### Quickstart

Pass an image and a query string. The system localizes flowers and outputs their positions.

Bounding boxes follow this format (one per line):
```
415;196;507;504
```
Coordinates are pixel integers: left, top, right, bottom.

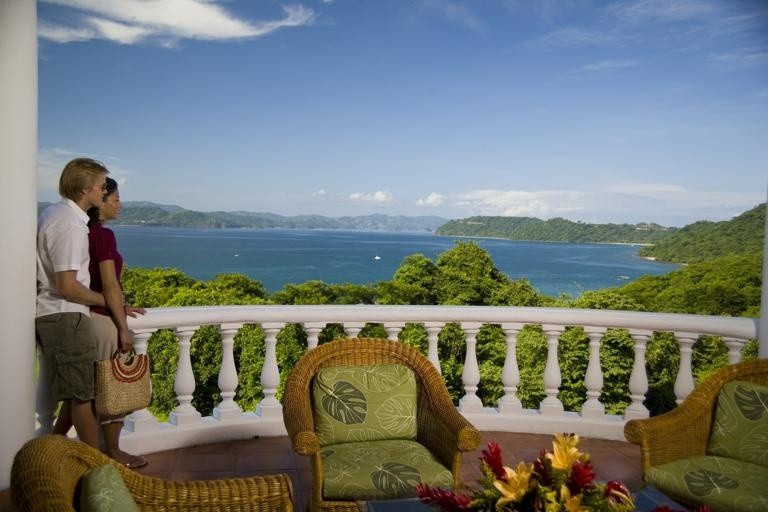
416;430;639;512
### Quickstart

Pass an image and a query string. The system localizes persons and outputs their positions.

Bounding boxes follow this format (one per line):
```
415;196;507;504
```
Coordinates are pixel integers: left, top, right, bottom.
35;157;129;450
86;174;149;470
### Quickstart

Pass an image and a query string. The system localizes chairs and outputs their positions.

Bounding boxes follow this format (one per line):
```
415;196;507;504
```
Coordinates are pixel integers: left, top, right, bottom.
11;435;295;511
625;358;766;512
283;336;482;506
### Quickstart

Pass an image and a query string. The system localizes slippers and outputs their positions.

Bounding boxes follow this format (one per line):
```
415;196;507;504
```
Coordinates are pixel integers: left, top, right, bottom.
110;454;149;469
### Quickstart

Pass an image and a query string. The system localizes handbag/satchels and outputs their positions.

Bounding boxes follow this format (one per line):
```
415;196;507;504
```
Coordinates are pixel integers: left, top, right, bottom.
93;346;153;417
